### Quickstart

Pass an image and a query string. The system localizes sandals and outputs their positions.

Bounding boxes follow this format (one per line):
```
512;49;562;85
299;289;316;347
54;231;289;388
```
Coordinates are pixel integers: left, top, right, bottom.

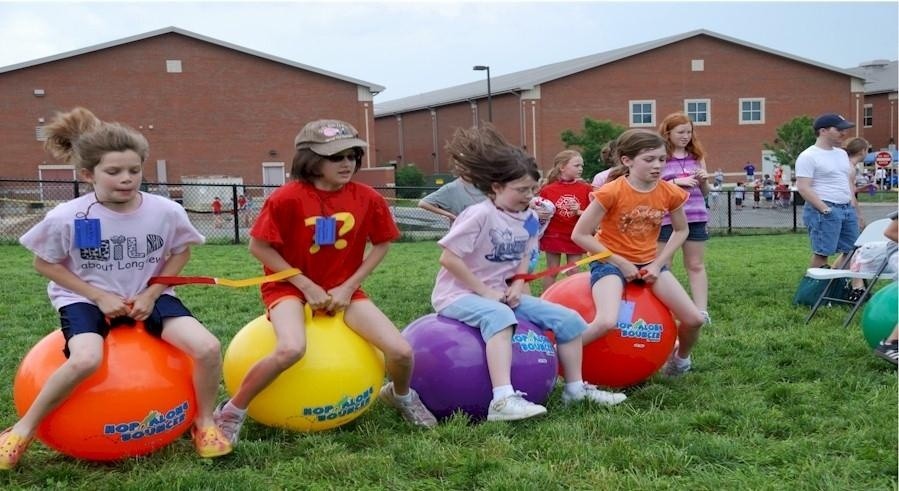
0;426;38;473
186;420;233;460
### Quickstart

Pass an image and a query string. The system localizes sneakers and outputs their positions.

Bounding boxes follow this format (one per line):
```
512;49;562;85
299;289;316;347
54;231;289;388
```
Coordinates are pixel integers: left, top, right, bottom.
664;340;692;379
700;311;713;329
485;391;548;423
847;286;870;304
874;333;898;367
377;382;439;430
559;381;628;410
212;399;250;446
735;206;762;210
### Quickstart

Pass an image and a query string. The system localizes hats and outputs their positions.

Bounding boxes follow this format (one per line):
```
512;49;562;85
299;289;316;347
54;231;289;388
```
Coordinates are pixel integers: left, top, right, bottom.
812;113;857;131
293;119;369;159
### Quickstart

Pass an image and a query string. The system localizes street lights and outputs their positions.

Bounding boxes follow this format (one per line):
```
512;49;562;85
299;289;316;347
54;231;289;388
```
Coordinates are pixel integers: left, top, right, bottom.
388;160;399;199
472;64;495;128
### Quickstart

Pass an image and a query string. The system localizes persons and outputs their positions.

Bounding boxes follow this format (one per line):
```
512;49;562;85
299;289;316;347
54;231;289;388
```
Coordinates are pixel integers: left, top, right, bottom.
209;194;254;231
0;106;233;470
539;149;596;291
592;139;630;188
212;118;440;451
430;121;628;422
554;130;705;379
418;155;490;228
656;112;713;324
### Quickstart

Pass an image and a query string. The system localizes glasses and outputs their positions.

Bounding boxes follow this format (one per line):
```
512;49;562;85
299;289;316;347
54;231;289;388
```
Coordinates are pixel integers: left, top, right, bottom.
502;183;541;198
318;151;360;165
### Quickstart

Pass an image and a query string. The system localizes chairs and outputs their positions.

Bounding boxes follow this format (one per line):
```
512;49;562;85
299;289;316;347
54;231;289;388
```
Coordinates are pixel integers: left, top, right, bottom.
804;219;899;330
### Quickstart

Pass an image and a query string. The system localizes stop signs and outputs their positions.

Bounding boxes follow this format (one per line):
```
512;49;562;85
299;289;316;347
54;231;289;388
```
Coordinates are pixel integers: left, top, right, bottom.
876;151;893;170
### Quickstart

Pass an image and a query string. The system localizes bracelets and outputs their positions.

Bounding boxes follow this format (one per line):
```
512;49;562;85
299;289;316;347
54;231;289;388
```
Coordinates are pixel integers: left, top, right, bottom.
670;177;676;185
